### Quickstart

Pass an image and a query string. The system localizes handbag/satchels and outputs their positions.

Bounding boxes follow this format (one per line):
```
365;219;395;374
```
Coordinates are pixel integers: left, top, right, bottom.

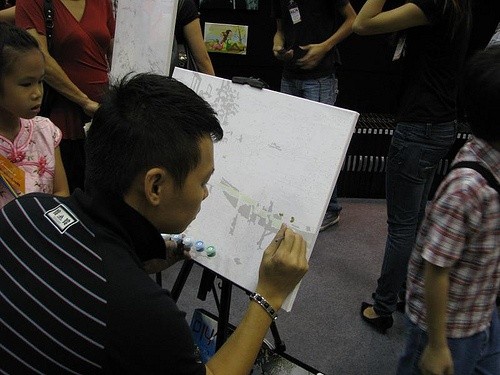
172;44;197;77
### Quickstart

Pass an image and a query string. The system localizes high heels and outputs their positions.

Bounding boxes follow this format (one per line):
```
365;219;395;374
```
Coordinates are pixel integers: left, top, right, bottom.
373;292;406;313
360;301;394;333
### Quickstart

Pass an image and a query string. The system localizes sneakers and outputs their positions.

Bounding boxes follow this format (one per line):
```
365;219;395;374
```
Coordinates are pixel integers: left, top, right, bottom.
319;209;339;231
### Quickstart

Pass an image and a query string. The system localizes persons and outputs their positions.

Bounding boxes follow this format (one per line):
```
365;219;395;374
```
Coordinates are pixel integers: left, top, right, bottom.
14;0;116;194
0;4;15;23
0;22;73;211
168;0;216;78
352;1;473;334
0;69;309;375
395;46;500;375
272;0;357;231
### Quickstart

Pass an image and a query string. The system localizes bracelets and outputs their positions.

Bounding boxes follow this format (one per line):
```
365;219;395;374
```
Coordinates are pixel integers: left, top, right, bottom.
249;292;279;323
